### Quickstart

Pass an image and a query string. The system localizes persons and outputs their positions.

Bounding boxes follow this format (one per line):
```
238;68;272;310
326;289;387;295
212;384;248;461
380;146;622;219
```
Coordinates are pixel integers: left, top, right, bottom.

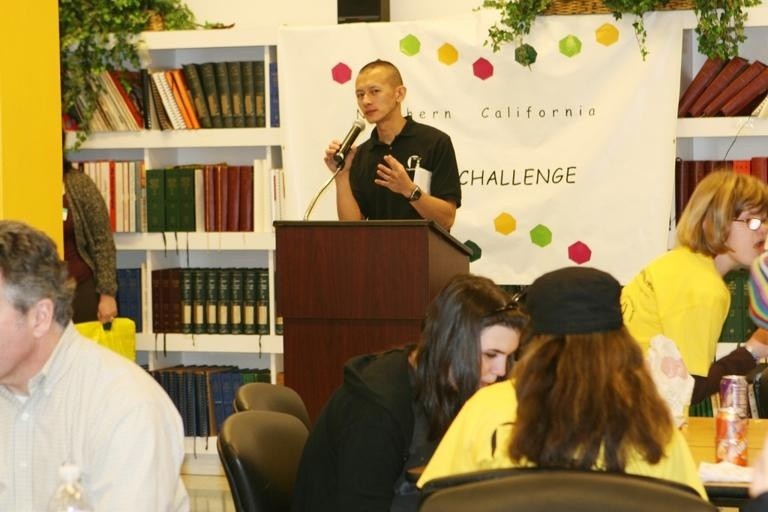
323;60;461;234
62;159;118;331
415;268;710;506
618;172;768;433
0;218;189;512
292;273;526;512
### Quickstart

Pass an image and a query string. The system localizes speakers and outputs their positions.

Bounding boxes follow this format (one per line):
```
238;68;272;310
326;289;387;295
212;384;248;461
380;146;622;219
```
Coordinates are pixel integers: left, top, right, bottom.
338;0;390;24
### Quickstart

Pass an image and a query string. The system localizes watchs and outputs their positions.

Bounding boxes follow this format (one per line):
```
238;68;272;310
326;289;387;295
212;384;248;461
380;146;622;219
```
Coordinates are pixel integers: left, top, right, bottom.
404;184;422;202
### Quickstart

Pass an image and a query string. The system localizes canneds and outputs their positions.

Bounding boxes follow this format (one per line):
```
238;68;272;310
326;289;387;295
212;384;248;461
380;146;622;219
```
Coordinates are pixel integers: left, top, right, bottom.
714;409;750;468
721;374;747;410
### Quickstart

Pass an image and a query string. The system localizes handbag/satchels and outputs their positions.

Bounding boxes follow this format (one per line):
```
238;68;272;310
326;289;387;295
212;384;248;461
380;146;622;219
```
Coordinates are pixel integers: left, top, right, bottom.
74;318;136;361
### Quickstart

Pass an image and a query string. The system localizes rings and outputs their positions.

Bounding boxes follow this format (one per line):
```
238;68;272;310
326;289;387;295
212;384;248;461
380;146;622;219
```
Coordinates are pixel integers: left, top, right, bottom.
110;317;114;320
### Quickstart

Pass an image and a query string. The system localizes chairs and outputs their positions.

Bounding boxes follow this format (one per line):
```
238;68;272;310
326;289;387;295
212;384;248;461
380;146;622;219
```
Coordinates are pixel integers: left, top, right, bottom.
229;381;316;437
215;410;311;511
415;466;722;511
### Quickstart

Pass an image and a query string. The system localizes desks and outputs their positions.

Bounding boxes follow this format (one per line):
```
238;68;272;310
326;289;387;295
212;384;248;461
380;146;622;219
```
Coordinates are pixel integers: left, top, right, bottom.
406;413;768;508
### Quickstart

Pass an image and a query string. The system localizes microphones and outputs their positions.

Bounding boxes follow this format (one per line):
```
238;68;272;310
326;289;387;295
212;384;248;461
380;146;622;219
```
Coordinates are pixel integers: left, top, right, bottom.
334;120;366;167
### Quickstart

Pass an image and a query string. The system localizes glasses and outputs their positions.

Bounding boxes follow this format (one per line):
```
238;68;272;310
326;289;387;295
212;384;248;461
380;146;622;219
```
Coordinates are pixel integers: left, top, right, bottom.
733;215;765;231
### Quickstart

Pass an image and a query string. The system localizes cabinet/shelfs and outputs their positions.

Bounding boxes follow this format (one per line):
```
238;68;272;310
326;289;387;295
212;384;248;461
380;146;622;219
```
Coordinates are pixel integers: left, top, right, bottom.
62;27;281;474
669;7;768;418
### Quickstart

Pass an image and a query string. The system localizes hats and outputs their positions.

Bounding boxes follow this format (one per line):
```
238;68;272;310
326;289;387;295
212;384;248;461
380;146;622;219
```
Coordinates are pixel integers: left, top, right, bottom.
525;267;624;334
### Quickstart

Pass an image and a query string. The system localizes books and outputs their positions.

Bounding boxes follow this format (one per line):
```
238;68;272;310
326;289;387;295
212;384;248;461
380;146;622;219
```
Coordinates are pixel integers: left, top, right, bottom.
677;57;768;420
141;364;270;437
62;60;266;134
118;267;267;335
61;157;268;232
